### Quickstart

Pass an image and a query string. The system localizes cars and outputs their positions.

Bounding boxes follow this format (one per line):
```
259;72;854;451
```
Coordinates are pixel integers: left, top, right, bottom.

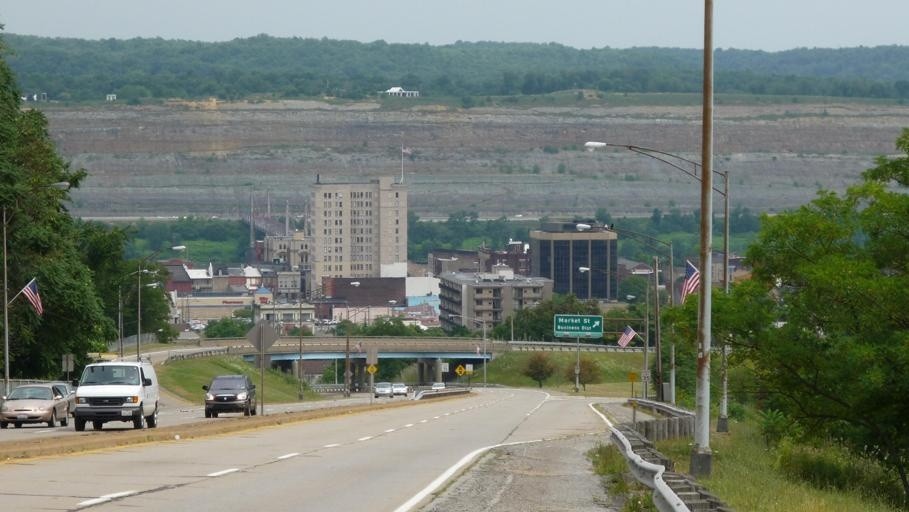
373;382;394;398
202;375;255;418
392;383;408;396
0;383;76;428
431;383;445;390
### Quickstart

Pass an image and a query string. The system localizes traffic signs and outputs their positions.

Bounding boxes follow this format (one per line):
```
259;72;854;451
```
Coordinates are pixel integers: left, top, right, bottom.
553;314;604;339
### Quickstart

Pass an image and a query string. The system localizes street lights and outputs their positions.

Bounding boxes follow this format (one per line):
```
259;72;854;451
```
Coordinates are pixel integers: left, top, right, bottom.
299;281;360;400
119;245;185;362
585;141;729;432
448;314;486;389
344;300;397;398
576;223;675;405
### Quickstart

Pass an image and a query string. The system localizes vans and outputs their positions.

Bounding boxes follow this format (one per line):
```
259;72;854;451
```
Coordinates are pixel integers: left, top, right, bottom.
74;362;159;431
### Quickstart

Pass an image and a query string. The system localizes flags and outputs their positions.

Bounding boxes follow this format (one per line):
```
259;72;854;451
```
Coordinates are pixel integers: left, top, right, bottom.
22;281;43;316
618;327;636;348
680;262;700;305
